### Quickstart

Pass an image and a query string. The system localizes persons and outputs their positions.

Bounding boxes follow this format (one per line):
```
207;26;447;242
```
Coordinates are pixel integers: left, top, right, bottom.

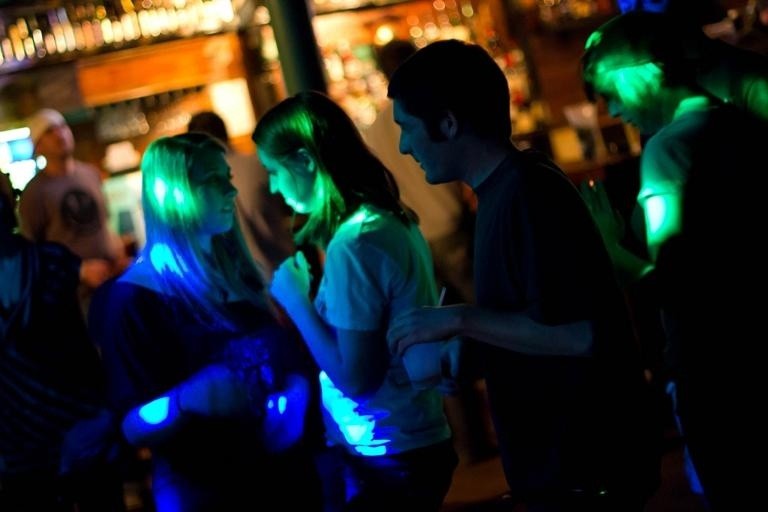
382;39;668;510
88;130;350;512
1;190;130;511
577;8;768;510
13;107;118;266
251;91;459;510
185;109;278;325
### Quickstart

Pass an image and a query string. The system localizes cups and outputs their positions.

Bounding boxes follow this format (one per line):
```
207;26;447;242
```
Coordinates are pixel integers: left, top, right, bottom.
402;340;443;392
